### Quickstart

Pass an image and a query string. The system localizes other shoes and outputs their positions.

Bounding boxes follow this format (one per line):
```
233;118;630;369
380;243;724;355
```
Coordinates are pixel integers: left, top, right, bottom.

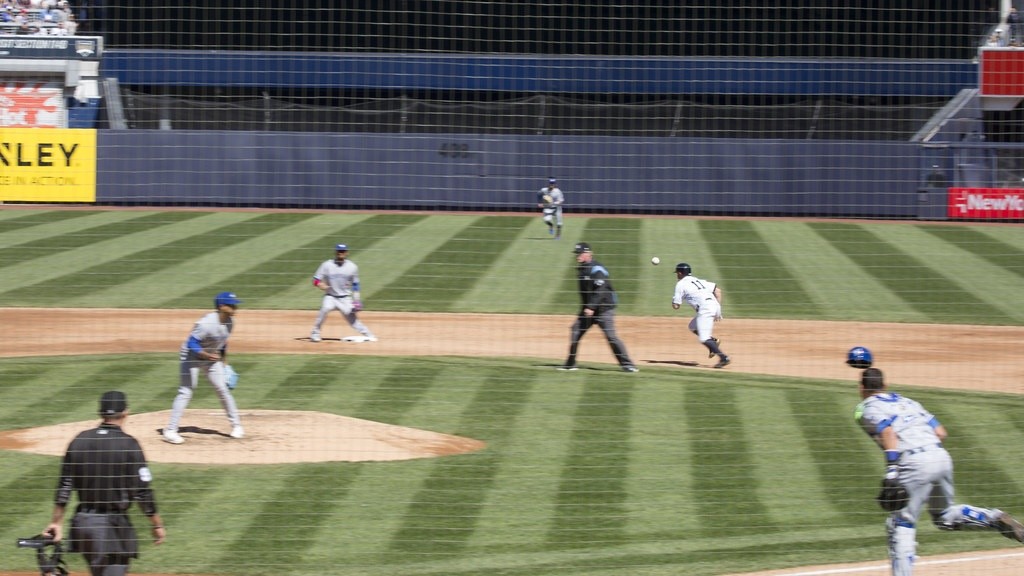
556;362;579;371
997;514;1024;542
366;336;377;342
715;356;730;369
626;367;639;372
709;338;720;359
311;330;321;342
230;426;245;439
162;428;185;443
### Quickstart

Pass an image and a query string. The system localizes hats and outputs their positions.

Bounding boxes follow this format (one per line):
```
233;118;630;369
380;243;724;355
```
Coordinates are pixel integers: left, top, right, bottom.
337;245;347;251
549;177;557;183
100;391;125;415
215;291;241;305
673;264;691;273
571;242;593;252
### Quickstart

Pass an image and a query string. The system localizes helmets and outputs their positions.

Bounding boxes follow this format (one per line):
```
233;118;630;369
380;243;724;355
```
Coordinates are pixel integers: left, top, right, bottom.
846;347;871;367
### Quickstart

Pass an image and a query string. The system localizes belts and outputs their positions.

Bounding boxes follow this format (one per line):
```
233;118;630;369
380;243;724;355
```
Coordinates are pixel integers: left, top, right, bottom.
908;442;943;455
696;298;713;312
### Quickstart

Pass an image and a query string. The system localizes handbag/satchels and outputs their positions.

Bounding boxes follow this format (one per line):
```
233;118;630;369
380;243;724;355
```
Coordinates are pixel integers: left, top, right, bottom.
106;515;138;559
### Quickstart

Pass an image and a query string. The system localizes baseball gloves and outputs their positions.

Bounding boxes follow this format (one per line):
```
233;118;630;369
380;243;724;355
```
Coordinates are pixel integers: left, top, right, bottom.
225;364;238;391
878;479;910;511
543;194;554;204
15;534;62;572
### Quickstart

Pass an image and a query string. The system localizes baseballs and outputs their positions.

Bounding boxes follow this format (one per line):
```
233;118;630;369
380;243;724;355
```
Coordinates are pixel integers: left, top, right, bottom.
652;257;660;264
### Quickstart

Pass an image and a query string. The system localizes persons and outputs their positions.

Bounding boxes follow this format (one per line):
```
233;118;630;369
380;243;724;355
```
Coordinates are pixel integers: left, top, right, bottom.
672;263;731;369
559;242;639;372
987;7;1024;48
0;0;78;37
40;391;167;576
537;176;565;240
854;367;1024;576
162;292;244;444
310;243;377;342
